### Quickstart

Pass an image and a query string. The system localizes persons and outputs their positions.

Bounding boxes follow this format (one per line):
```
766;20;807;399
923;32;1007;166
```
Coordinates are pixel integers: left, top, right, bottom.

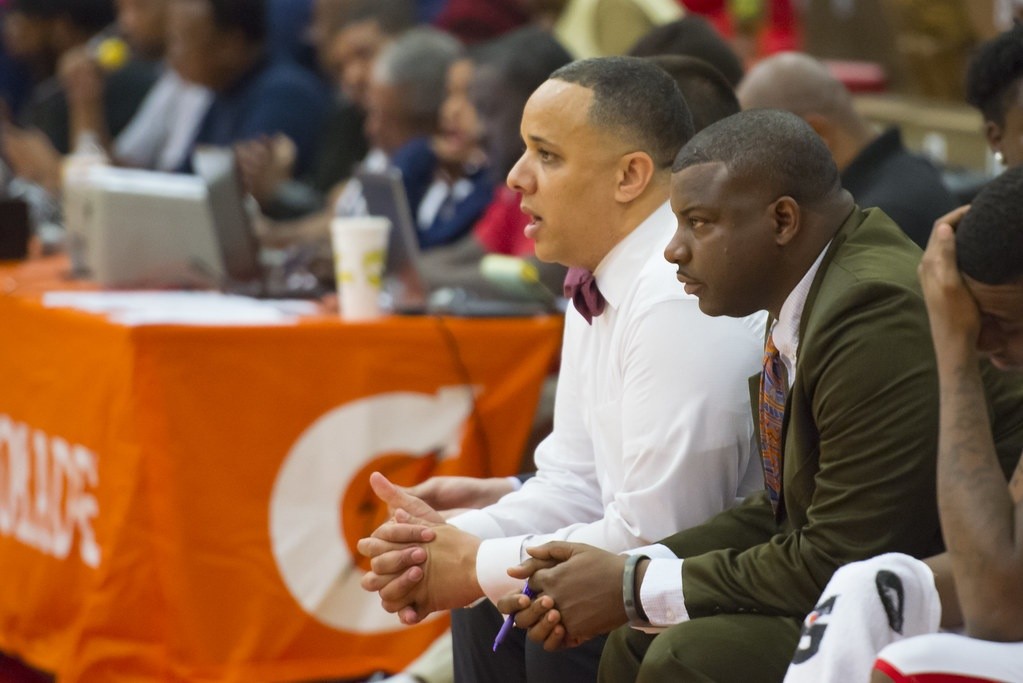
781;165;1023;683
736;52;957;251
968;26;1023;168
357;55;769;683
629;20;747;86
496;104;1023;683
0;0;573;313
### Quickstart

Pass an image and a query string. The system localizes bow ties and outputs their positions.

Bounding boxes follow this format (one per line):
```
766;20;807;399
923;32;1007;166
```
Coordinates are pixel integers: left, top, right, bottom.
563;267;606;325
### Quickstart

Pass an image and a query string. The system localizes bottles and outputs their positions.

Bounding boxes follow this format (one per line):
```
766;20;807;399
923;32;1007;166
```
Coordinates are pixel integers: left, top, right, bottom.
62;129;108;277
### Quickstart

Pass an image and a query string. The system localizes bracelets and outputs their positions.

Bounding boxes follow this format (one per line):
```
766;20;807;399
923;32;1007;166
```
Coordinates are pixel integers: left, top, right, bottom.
622;555;650;626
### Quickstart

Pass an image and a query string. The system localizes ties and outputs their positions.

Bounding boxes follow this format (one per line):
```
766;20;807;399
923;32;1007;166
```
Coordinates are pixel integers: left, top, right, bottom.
759;320;793;526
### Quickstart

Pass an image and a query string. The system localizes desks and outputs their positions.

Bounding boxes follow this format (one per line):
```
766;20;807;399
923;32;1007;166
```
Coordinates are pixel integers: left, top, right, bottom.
2;256;561;683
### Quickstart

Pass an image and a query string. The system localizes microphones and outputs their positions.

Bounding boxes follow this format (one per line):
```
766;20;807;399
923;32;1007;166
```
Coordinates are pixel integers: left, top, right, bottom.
32;38;132;105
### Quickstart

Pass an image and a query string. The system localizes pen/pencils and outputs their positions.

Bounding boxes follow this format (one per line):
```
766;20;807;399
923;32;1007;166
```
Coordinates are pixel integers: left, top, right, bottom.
492;583;535;652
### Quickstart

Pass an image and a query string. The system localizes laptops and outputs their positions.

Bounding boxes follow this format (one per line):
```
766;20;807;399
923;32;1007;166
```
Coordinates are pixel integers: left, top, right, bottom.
354;163;552;314
196;144;329;297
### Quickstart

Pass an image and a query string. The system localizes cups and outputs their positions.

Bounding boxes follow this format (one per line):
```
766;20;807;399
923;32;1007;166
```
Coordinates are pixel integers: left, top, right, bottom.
327;215;393;324
